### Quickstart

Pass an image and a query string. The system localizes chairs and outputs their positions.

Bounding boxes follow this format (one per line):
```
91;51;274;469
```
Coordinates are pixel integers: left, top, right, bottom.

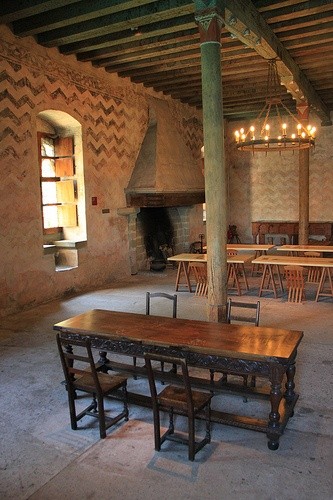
210;297;260;403
144;353;214;462
188;262;207;297
283;266;306;304
305;252;321;284
56;332;129;440
133;291;178;386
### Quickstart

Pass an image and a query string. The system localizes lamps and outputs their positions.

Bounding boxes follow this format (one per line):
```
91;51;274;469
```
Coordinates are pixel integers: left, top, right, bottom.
234;58;317;155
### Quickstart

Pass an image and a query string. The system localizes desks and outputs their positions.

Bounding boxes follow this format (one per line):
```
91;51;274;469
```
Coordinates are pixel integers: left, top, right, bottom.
53;308;304;450
167;244;333;302
